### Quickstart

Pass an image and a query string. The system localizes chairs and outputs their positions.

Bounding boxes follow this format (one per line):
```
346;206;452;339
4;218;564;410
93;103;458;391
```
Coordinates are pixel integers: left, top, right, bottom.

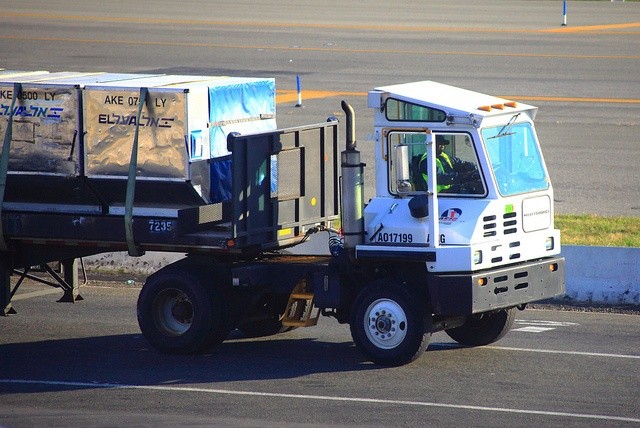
411;155;426;192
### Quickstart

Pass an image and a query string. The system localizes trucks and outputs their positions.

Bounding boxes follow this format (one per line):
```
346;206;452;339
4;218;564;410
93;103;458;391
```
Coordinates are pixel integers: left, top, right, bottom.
0;68;566;367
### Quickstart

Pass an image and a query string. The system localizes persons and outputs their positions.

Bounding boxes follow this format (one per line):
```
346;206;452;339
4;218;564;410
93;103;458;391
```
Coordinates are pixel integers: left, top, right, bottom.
418;136;471;194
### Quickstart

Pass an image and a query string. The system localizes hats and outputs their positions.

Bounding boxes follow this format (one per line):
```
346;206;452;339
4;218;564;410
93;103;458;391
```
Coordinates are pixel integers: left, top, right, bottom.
437;136;449;145
